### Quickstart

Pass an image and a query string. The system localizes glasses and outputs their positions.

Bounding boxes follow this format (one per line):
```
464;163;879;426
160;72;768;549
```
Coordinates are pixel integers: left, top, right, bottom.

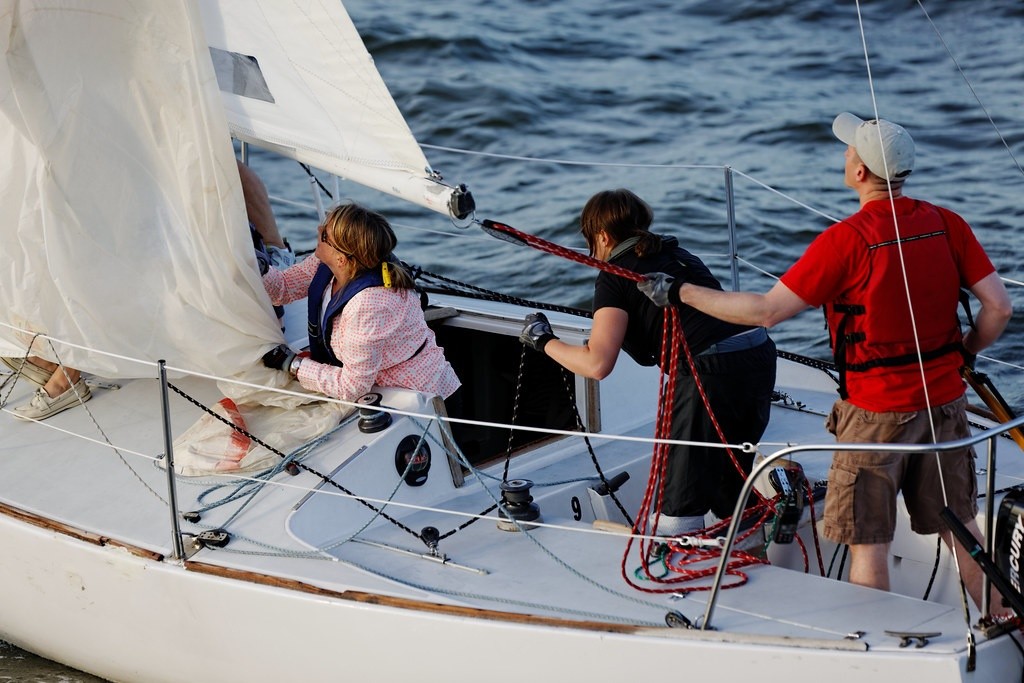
322;222;353;258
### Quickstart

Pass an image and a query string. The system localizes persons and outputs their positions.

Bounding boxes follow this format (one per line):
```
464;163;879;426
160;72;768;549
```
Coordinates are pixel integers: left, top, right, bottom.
632;111;1014;621
256;201;482;466
236;155;290;338
516;188;777;564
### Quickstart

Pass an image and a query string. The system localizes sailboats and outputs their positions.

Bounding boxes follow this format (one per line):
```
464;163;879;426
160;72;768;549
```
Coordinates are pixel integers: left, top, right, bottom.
0;2;1024;679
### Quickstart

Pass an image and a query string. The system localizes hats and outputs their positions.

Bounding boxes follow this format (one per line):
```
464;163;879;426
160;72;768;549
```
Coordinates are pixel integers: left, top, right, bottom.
832;112;914;182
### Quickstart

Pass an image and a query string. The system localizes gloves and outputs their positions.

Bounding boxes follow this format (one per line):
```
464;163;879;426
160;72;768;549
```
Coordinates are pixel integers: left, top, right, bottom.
520;312;560;351
637;273;682;305
262;345;297;373
955;336;976;370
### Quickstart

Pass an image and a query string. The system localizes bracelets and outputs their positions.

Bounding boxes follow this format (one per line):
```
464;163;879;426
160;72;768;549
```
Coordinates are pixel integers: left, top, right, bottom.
290;355;303;375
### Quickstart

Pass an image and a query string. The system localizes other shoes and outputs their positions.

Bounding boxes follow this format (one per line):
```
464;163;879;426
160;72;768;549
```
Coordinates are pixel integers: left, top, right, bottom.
13;377;92;421
1;357;52;388
